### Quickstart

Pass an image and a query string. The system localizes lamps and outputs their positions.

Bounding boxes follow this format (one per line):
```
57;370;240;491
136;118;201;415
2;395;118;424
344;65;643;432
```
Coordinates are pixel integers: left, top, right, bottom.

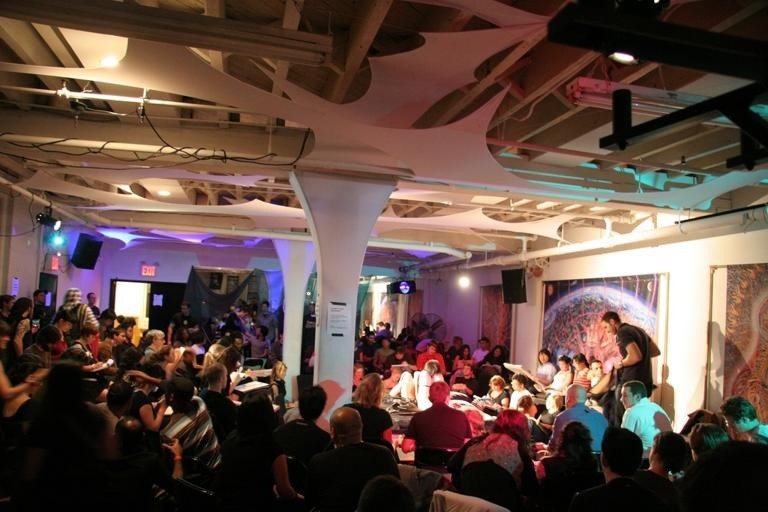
36;199;64;233
0;0;336;71
558;72;766;131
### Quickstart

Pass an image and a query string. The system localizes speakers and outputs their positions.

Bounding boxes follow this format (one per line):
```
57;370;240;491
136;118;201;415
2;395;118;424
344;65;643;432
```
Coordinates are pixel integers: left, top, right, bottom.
70;233;104;270
208;271;224;290
501;268;527;304
390;280;416;295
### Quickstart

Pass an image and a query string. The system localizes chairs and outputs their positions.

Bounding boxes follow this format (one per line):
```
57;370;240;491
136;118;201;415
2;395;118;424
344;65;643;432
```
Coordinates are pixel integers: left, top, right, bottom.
10;319;650;511
